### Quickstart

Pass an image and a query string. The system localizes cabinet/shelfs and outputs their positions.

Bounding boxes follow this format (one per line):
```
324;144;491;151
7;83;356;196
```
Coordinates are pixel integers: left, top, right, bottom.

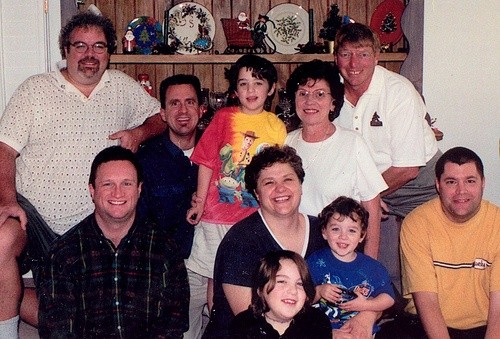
60;0;424;113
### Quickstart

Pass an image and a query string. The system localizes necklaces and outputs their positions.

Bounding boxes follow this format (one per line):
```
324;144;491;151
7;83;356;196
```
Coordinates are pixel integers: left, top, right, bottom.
265;314;291;323
296;126;329;170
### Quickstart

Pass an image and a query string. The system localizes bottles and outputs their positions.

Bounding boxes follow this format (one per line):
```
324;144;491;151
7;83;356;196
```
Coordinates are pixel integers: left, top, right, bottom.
125;26;136;54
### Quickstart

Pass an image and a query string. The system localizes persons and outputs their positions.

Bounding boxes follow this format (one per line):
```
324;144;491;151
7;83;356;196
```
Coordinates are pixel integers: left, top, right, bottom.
0;13;167;339
213;145;406;339
37;146;190;339
227;250;332;339
399;147;500;339
331;23;443;296
134;74;208;339
283;59;389;260
306;196;395;339
186;55;287;315
252;14;268;53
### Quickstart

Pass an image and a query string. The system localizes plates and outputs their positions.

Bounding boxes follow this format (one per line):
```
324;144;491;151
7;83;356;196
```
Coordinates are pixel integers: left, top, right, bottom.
261;3;309;54
125;16;163;55
162;2;216;55
370;0;405;46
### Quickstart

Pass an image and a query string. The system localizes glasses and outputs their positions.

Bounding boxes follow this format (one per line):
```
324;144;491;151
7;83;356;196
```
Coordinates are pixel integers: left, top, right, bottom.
295;90;332;99
69;41;109;54
